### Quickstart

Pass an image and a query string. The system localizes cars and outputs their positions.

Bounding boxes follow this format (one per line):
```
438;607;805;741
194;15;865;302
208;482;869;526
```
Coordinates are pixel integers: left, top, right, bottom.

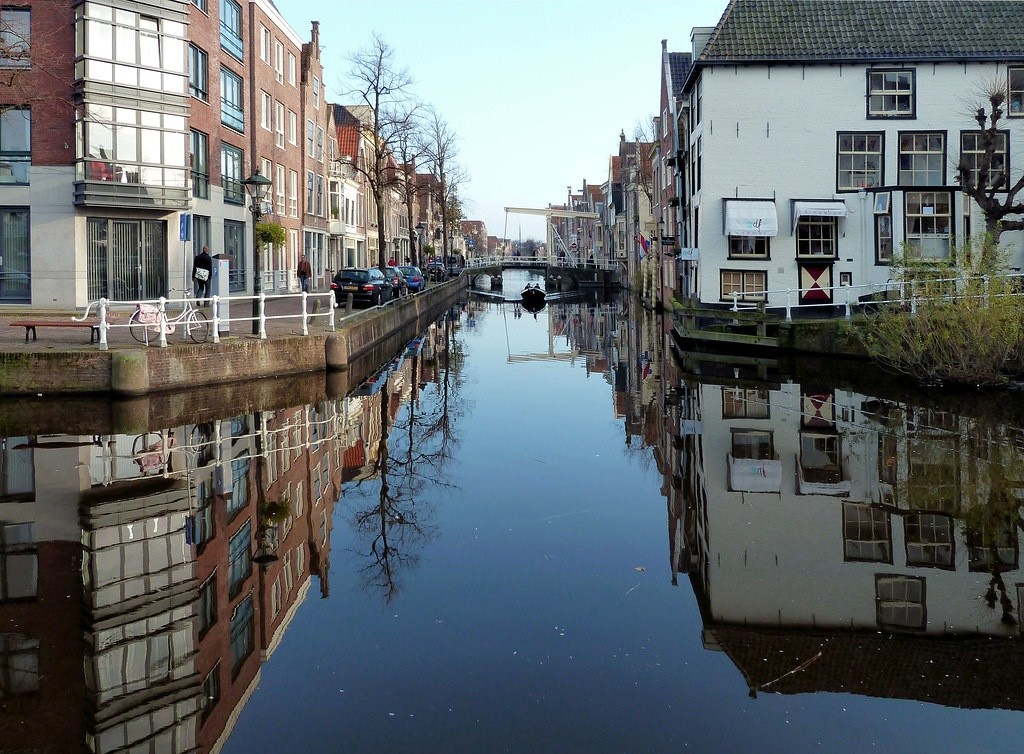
399;266;424;293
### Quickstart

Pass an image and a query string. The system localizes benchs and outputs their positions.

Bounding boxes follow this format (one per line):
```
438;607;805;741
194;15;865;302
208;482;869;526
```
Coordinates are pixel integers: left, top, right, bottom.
8;320;115;344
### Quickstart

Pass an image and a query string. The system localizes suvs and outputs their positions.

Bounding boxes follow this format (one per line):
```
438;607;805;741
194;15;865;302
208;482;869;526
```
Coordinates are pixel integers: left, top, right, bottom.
427;263;447;282
330;265;393;308
377;267;408;299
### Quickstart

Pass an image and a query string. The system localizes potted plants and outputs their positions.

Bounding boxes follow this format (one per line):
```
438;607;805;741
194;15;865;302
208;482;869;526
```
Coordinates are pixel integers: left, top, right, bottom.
667;197;679;207
331;206;338;218
254;220;287;254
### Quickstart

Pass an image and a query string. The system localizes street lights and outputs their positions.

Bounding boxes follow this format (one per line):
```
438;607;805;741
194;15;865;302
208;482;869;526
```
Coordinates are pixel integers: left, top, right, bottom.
244;166;272;334
415;221;424;270
247;412;279;573
448;236;454;278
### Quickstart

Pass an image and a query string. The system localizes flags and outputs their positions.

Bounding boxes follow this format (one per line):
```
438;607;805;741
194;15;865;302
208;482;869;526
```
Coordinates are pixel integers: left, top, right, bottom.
639;235;650;260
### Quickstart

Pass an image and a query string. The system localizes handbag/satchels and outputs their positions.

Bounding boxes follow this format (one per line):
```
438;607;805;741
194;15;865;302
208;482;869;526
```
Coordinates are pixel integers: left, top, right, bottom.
195;267;210;281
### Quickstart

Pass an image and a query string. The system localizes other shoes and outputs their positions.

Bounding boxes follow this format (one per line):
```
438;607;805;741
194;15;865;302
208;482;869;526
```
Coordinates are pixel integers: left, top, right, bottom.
197;301;200;306
204;304;210;308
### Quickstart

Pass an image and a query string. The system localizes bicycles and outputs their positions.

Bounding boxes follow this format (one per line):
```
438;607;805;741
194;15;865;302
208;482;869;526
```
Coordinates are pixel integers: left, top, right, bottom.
128;287;208;345
132;422;212;474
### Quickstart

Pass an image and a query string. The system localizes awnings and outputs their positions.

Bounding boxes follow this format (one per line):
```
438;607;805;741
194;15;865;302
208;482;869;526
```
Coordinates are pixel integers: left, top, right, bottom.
792;201;847;237
725;200;777;237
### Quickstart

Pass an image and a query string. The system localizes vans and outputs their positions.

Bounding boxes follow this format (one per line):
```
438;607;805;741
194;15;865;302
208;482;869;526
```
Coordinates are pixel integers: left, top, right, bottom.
443;256;460;274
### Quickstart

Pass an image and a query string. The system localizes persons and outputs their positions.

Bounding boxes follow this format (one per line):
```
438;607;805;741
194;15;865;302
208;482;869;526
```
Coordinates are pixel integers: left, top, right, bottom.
405;256;411;266
427;256;442;263
557;248;565;267
192;246;213;307
298;255;312;293
512;249;521;266
532;249;536;265
371;260;378;267
388;257;396;267
